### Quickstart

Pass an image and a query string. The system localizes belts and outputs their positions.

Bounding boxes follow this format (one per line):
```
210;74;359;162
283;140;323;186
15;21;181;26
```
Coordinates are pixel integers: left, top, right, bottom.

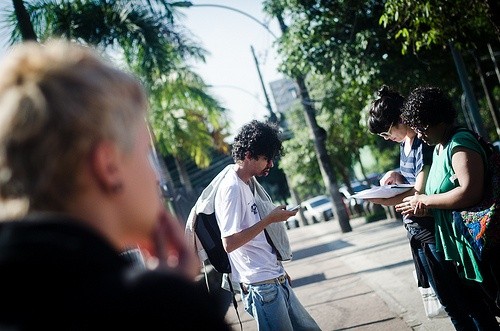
251;275;286;285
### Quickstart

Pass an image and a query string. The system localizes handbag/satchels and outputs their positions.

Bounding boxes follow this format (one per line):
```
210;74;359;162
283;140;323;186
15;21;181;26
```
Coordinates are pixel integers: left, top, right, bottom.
413;269;448;320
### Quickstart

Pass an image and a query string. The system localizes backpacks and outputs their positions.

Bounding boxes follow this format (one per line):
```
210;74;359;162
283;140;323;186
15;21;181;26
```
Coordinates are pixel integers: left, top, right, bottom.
447;129;500;255
194;213;243;331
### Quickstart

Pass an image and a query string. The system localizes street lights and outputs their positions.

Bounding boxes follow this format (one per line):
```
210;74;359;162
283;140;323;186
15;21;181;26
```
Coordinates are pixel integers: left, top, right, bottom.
171;1;282;42
282;177;385;229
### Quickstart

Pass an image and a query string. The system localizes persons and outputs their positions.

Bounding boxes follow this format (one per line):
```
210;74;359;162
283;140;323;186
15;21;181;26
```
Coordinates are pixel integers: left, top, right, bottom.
0;37;233;331
213;121;323;331
361;85;500;331
395;87;500;331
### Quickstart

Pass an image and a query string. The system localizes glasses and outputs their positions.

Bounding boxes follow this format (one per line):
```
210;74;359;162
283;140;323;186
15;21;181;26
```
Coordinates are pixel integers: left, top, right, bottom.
377;121;393;137
414;123;430;133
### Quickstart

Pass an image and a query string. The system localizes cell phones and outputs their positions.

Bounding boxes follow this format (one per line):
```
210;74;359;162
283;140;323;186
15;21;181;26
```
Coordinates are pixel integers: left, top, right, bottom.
287;204;301;211
